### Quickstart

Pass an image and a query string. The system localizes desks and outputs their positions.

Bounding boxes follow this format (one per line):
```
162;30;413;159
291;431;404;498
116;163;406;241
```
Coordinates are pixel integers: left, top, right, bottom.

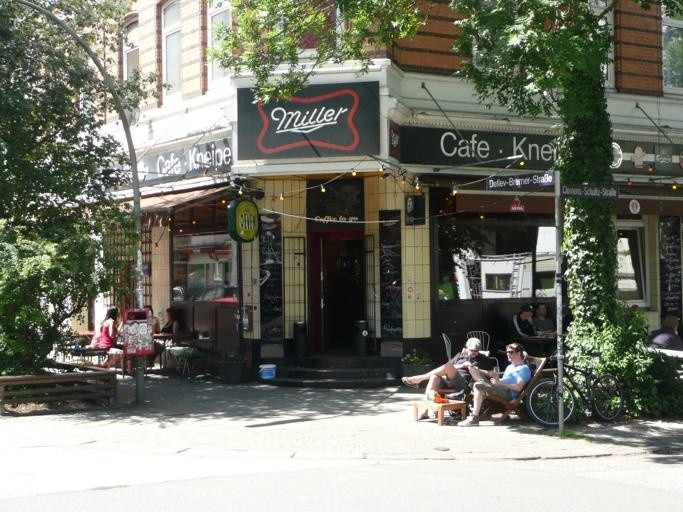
153;331;173;369
416;397;468;424
513;335;555;353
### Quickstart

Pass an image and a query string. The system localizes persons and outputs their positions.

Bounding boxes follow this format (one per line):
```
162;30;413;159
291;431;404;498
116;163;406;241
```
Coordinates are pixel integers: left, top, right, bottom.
514;304;541;356
647;316;683;351
145;308;178;366
401;338;493;420
626;305;640;324
456;344;531;426
91;309;135;375
144;305;160;332
531;304;557;336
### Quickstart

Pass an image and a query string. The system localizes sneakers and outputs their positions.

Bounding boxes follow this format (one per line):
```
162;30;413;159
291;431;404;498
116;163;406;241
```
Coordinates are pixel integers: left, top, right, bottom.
458;413;479;426
474;379;492;391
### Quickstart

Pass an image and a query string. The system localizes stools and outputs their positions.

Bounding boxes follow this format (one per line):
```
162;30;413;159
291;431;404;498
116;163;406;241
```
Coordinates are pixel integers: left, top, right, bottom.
172;347;199;376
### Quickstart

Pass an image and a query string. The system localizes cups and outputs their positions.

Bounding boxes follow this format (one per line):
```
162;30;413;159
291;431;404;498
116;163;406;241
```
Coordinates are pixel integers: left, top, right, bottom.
494;367;499;380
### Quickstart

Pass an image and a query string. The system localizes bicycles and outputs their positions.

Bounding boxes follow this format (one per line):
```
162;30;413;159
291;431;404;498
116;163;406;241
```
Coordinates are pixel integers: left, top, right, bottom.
525;347;624;428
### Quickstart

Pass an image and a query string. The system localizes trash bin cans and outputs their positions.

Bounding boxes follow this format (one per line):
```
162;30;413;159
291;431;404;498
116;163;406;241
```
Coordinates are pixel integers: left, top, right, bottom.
293;320;306;357
354;320;368;357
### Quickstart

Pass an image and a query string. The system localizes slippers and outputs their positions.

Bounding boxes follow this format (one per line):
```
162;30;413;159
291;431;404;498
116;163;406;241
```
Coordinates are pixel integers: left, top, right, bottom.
418;414;429;420
401;377;419;389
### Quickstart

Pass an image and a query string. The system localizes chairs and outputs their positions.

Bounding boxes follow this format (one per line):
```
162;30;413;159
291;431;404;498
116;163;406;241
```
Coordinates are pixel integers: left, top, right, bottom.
442;330;547;421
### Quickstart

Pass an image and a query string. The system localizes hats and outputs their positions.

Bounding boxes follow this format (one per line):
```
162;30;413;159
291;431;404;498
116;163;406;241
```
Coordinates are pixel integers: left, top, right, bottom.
520;304;536;312
466;338;481;351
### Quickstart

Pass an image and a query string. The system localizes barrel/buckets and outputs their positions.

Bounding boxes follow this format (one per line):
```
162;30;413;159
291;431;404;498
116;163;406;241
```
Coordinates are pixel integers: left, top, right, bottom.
258;364;276;379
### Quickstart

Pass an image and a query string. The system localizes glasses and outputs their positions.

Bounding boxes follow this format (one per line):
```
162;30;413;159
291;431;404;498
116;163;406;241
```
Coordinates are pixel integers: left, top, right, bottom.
506;351;513;354
